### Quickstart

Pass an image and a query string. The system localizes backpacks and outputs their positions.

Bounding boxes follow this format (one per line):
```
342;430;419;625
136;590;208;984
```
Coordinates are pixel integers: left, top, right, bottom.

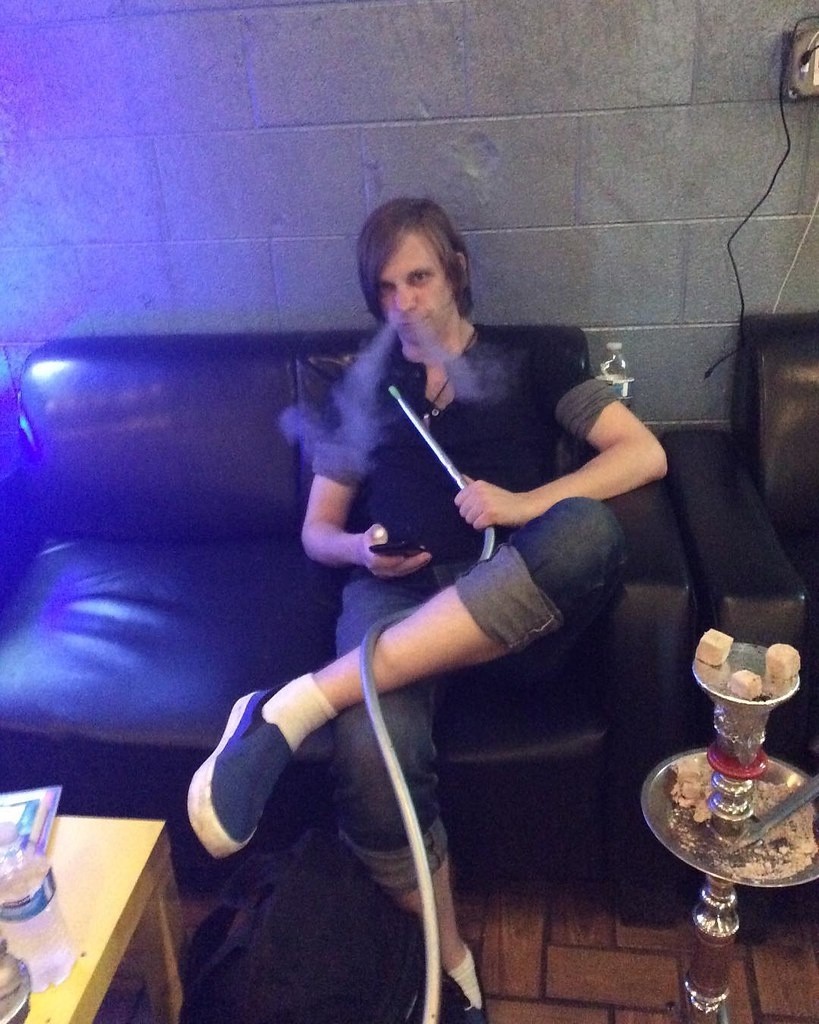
178;838;466;1024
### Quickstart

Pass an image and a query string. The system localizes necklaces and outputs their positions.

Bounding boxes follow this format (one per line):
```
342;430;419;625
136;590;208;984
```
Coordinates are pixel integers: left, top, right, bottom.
422;329;478;431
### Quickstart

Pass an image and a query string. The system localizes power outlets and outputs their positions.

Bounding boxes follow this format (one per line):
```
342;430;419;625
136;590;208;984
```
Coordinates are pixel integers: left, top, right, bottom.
787;27;819;100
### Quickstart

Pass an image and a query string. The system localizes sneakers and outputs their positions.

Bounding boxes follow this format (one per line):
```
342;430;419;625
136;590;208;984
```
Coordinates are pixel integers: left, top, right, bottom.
187;683;293;858
454;947;486;1024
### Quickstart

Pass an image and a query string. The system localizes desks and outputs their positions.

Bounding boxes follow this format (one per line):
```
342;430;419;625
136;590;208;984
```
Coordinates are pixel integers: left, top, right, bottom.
1;815;191;1024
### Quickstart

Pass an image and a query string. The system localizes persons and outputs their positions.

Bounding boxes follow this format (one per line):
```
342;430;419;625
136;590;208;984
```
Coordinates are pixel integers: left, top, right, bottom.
186;198;668;1024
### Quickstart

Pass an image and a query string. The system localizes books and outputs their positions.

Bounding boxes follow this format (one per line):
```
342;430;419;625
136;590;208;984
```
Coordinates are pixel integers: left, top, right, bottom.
0;783;62;856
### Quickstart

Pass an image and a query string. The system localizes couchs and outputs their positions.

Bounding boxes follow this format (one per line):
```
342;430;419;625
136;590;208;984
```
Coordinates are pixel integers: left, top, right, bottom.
0;327;691;923
659;312;818;917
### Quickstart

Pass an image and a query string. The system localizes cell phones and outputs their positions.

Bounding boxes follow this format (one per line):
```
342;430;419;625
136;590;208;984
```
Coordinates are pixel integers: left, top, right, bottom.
369;540;426;556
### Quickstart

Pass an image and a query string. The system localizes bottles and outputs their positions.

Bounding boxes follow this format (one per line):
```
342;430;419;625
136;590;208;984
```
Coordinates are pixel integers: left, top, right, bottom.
595;342;635;412
0;823;73;995
